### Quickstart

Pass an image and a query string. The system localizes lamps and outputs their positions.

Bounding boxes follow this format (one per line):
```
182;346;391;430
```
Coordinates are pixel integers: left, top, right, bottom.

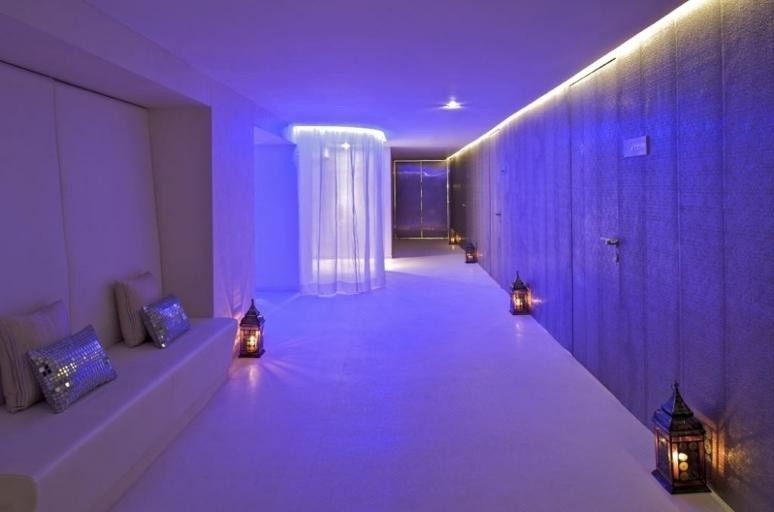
509;270;530;316
448;228;458;245
650;379;710;496
464;241;477;265
237;297;266;360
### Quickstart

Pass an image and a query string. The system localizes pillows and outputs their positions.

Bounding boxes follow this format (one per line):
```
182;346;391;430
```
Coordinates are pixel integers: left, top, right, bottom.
0;296;73;414
138;293;191;351
24;324;117;413
112;270;162;348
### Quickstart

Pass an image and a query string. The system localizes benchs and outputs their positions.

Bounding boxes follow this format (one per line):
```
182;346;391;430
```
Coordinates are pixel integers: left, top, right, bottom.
0;314;236;512
